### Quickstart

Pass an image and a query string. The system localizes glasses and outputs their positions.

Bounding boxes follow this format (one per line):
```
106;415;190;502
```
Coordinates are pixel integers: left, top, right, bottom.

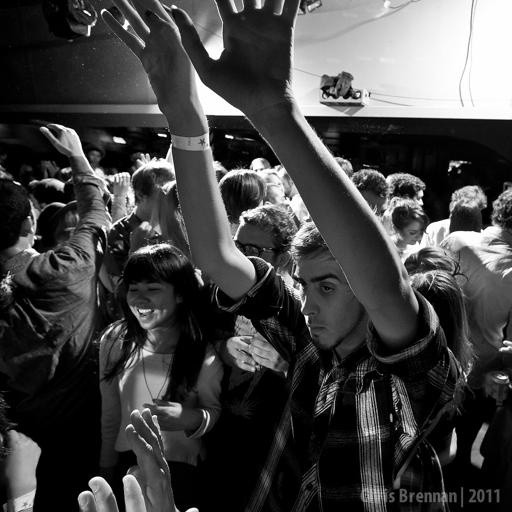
233;240;275;257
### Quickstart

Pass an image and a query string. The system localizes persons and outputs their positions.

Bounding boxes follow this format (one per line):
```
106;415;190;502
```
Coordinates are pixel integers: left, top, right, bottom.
98;0;466;512
